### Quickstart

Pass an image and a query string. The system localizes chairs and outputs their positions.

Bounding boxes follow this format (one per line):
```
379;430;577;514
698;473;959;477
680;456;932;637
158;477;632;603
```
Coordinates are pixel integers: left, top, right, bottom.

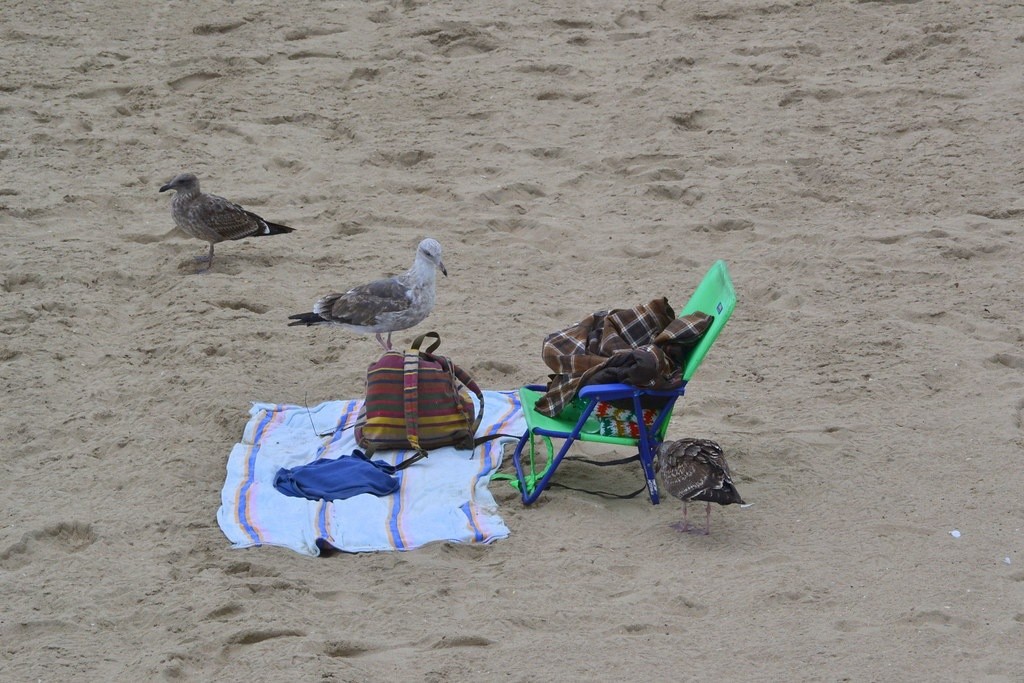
512;260;739;510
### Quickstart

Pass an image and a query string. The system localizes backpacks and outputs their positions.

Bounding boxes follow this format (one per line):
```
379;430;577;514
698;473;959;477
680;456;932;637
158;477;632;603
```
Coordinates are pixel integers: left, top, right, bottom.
353;331;523;460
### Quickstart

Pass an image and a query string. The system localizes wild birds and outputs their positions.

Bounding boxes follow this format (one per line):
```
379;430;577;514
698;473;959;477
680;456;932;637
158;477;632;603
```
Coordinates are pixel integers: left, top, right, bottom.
287;238;449;351
160;171;295;272
656;435;746;538
